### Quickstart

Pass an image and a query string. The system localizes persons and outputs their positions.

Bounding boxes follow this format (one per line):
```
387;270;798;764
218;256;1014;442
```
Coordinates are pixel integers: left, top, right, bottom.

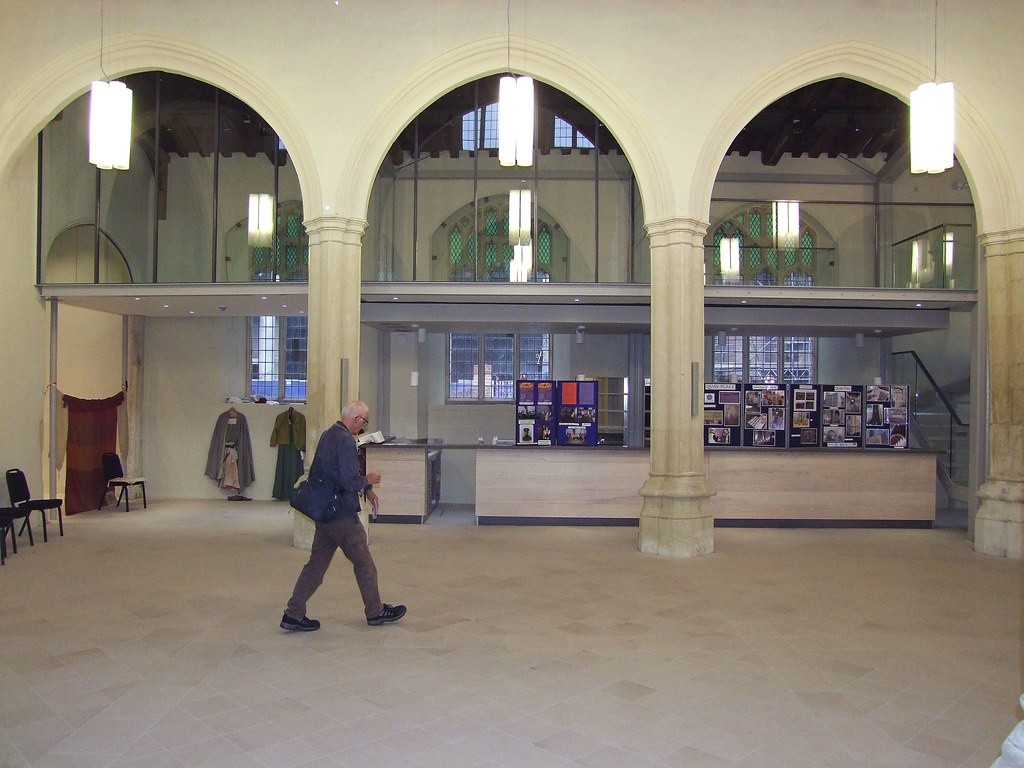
753;392;760;407
713;433;727;442
522;428;531;441
280;400;407;632
763;391;784;405
543;427;550;439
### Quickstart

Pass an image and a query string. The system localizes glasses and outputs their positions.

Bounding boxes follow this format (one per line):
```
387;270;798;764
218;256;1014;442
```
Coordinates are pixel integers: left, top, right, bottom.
356;415;369;426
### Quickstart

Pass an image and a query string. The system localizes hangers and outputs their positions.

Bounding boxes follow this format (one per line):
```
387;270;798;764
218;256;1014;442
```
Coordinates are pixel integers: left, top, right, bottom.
278;402;307;419
219;400;247;420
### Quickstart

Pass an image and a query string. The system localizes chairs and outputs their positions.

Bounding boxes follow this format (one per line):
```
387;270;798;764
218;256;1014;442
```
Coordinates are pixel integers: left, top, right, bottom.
0;468;63;565
98;452;147;513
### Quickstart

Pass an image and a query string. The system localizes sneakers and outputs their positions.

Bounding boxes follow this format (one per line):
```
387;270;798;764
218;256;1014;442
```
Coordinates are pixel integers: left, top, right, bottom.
280;614;320;631
367;604;407;626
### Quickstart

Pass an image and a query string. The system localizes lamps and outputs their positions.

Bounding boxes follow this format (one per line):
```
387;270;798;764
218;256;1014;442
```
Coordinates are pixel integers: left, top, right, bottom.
514;237;533;271
510;259;528;282
248;193;276;249
88;77;133;171
874;330;882;386
718;330;727;347
910;0;956;175
855;333;865;348
497;0;535;247
418;328;426;343
576;329;584;344
410;324;419;387
720;201;801;272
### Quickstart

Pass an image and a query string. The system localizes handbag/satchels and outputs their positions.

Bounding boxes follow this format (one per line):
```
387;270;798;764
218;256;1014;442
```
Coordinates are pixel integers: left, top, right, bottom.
290;476;341;524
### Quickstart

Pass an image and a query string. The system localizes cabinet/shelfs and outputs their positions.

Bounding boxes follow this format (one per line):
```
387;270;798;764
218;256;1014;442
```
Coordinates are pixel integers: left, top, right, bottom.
362;434;441;524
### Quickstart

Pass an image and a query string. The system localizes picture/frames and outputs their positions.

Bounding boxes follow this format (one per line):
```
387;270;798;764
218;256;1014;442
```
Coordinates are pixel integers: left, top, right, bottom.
704;385;908;448
